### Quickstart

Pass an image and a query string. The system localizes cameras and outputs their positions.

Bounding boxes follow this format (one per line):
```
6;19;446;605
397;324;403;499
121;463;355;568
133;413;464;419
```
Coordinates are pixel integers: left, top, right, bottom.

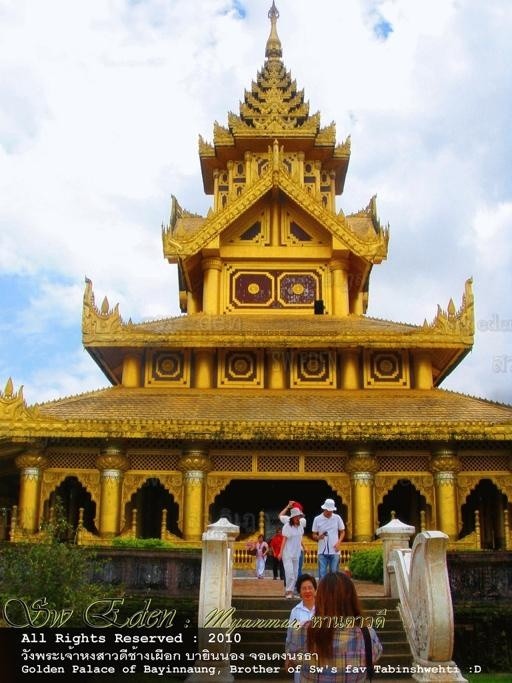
323;531;329;536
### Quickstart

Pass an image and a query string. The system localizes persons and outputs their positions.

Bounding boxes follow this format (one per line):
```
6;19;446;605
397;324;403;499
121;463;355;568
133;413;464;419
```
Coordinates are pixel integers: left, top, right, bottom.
285;570;383;678
256;499;345;590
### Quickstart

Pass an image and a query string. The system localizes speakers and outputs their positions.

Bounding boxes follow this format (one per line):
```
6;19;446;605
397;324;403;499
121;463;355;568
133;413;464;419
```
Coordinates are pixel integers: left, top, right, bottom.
314;300;323;314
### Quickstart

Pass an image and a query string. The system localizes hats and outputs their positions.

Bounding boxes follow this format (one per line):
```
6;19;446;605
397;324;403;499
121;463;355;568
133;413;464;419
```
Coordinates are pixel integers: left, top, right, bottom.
288;503;306;519
320;498;337;511
